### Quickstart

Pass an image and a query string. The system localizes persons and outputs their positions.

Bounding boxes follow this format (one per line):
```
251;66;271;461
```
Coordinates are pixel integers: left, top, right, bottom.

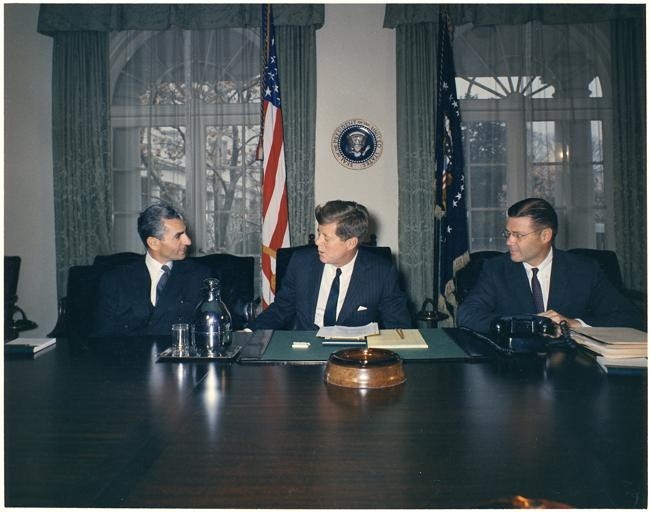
88;203;214;337
236;200;413;333
455;197;644;332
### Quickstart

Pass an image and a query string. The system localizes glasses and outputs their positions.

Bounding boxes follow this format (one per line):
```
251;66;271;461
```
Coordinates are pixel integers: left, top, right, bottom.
503;227;539;240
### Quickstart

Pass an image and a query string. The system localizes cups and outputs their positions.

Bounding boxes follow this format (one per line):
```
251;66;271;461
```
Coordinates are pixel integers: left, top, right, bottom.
171;323;190;357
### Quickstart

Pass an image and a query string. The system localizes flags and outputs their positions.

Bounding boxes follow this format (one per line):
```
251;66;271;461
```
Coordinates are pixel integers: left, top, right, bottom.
256;4;291;310
433;5;471;329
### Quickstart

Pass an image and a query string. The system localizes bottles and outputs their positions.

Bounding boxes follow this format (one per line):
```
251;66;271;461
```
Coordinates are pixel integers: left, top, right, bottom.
190;278;233;358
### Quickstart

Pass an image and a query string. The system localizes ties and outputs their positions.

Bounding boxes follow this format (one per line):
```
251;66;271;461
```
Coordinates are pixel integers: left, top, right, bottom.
323;269;342;326
155;266;170;304
530;268;544;314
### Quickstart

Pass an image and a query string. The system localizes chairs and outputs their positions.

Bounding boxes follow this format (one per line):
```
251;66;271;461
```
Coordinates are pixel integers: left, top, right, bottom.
2;255;40;339
191;253;261;320
275;244;393;298
453;250;508;305
564;247;646;305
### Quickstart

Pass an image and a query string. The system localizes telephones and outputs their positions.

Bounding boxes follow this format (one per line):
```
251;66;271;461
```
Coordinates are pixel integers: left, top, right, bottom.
489;314;555;357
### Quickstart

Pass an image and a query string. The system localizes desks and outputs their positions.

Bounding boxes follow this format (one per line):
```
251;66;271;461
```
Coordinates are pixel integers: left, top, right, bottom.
4;330;650;508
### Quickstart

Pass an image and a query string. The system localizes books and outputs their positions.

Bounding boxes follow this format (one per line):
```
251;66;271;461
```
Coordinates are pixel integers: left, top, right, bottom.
316;322;429;350
4;336;57;354
571;325;647;366
